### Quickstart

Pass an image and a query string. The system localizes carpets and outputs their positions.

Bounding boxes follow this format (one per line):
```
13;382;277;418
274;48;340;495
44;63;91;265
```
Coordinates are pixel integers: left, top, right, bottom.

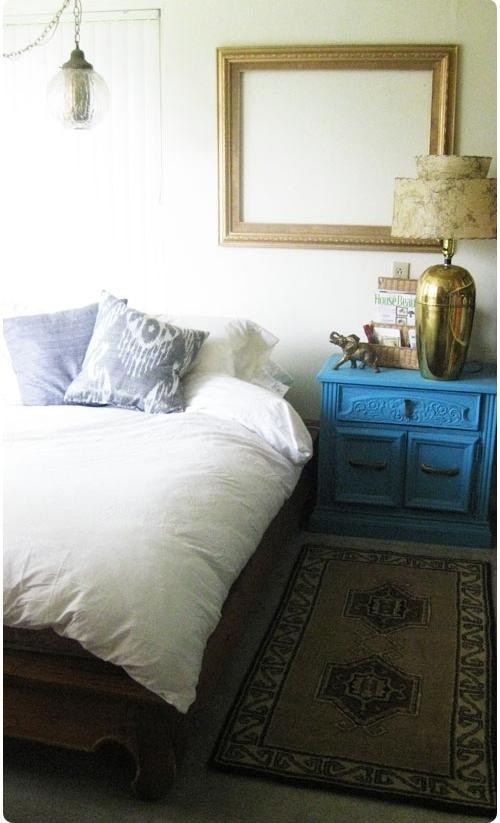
208;543;498;817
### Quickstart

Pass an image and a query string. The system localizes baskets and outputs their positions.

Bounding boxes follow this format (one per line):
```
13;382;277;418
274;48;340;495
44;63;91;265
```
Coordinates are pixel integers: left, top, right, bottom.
369;343;418;371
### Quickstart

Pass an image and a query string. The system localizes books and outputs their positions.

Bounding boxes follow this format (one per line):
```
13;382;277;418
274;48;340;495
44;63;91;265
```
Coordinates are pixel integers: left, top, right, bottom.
372;290;416;348
373;327;401;347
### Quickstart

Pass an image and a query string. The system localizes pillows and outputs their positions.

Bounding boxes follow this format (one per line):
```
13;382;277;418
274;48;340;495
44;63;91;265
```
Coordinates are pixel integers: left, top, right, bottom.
1;302;130;407
155;310;275;390
62;289;210;417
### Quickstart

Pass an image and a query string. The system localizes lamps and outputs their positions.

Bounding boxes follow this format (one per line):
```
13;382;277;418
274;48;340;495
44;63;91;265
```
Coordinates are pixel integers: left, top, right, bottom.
391;155;497;379
0;0;111;129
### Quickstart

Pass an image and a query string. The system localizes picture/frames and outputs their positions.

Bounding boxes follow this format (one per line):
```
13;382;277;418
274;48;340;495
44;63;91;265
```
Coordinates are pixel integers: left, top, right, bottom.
216;46;460;255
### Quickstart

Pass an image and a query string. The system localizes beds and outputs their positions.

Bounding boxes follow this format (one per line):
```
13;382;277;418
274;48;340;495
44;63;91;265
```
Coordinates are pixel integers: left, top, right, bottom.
5;394;320;802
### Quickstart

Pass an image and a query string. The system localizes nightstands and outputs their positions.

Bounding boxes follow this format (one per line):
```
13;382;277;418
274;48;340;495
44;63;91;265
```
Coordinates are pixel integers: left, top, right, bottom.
309;354;498;548
